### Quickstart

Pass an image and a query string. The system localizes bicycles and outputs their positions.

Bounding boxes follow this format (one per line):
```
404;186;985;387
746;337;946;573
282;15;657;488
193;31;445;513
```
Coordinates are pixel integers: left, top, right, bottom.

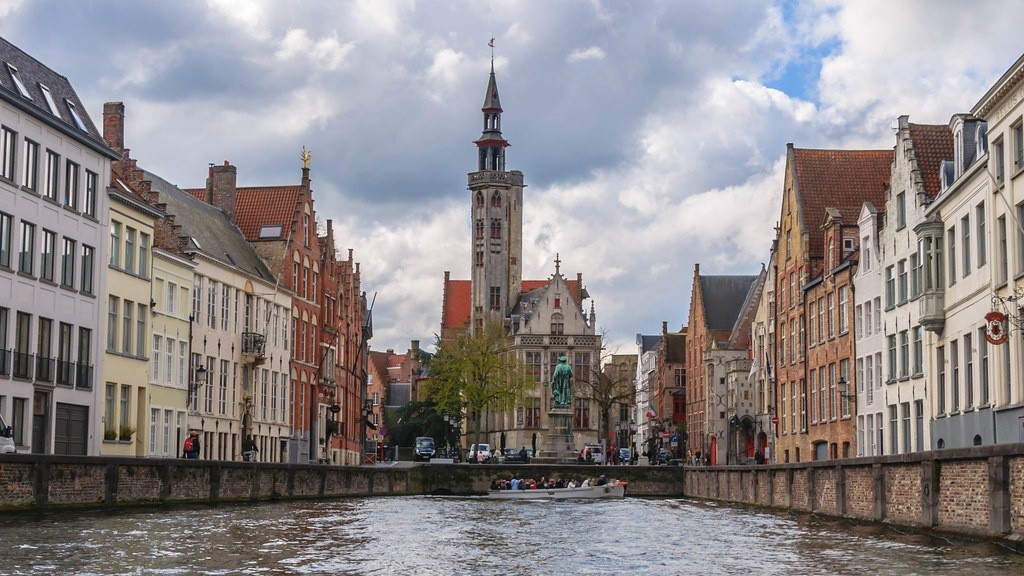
246;450;259;462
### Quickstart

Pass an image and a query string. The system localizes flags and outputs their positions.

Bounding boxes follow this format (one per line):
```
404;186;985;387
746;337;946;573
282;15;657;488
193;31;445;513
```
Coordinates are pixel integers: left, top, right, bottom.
766;353;771;376
646;403;657;418
748;357;760;381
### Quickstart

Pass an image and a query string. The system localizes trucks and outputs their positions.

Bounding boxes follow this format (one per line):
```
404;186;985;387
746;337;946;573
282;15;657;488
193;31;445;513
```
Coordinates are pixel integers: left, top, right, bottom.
414;436;434;457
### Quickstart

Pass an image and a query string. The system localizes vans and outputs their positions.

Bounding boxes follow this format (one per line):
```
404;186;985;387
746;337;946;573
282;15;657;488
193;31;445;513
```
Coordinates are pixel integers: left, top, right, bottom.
582;443;603;464
469;442;492;463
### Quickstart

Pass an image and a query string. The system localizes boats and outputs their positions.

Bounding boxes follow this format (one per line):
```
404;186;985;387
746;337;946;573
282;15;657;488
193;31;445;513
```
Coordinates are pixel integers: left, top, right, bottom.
486;481;627;500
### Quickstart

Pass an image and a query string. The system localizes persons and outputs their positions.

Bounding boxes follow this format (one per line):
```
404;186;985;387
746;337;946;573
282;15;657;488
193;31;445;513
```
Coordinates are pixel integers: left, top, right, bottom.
242;434;258;462
551;356;574;405
182;430;200;460
441;443;764;491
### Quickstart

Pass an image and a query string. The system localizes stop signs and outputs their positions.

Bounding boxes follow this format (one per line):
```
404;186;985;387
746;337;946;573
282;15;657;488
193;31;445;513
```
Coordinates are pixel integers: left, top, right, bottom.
772;416;779;424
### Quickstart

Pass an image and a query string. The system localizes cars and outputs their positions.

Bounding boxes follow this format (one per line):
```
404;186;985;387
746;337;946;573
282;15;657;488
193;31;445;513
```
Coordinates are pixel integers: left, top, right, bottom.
650;450;674;463
442;447;461;462
613;448;632;461
500;447;520;460
0;415;16;453
